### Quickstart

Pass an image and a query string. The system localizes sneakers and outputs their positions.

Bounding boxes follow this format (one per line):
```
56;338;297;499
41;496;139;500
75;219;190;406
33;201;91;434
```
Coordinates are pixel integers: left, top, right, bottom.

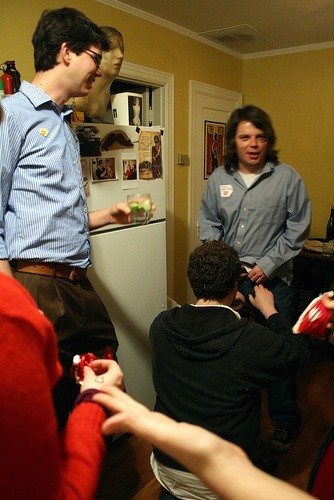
268;428;296;454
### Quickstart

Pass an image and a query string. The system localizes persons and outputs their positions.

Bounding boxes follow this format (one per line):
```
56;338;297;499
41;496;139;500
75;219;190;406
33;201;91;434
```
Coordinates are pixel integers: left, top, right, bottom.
0;8;141;500
65;25;125;120
0;103;334;500
95;135;161;181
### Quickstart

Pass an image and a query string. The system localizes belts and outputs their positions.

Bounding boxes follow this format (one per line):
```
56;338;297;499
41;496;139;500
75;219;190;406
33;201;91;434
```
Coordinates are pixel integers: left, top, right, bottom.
11;260;88;281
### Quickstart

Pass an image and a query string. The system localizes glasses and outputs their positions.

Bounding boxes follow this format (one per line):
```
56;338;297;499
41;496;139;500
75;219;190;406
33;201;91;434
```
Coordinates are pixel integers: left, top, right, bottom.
85;47;102;68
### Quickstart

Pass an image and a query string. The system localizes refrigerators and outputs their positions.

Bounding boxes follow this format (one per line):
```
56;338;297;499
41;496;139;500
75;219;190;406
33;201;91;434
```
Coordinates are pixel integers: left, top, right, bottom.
71;122;168;402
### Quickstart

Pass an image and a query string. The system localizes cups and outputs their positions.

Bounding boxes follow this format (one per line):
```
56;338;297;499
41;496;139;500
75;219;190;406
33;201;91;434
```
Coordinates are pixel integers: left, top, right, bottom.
322;243;333;256
127;188;151;224
329;240;334;254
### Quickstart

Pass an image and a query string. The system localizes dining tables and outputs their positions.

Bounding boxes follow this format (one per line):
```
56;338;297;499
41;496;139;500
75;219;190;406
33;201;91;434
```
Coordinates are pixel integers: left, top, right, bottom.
289;240;334;324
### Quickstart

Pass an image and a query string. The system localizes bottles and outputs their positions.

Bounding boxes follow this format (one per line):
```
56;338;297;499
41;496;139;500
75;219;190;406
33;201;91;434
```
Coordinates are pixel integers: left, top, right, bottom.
0;70;14;94
3;60;21;93
326;205;334;242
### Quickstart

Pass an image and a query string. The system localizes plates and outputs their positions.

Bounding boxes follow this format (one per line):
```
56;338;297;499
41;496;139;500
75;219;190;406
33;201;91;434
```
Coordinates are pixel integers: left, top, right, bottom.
302;241;322;252
